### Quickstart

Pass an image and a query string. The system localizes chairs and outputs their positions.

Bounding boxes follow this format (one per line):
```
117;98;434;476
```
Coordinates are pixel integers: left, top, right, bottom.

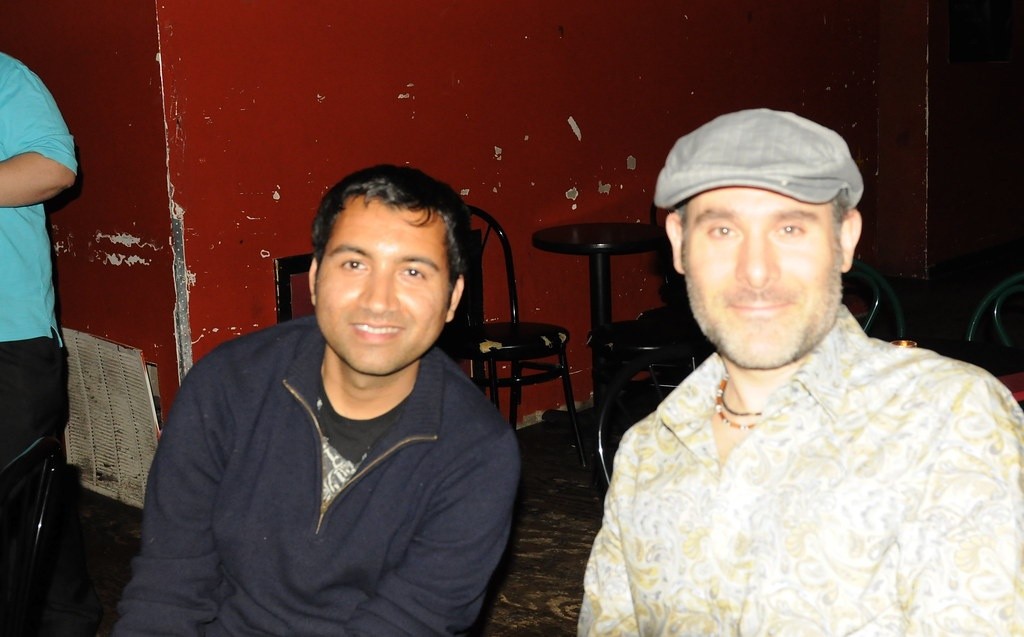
438;205;587;467
0;437;65;637
965;272;1024;348
841;259;905;342
587;204;718;494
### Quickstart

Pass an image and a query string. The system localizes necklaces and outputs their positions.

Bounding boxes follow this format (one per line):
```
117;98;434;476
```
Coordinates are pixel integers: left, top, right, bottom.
717;374;762;428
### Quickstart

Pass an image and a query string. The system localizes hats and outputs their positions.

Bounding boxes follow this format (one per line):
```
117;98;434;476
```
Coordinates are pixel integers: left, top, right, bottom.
653;109;865;209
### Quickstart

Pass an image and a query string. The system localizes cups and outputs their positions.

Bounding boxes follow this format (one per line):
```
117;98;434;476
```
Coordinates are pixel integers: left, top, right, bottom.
890;340;917;347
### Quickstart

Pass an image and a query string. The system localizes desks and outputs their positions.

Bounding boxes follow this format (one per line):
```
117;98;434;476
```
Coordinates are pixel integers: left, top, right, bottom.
889;338;1024;412
531;223;670;489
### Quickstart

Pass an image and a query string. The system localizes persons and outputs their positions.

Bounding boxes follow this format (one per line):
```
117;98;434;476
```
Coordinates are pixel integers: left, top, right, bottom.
109;164;519;637
576;108;1022;637
0;54;77;637
